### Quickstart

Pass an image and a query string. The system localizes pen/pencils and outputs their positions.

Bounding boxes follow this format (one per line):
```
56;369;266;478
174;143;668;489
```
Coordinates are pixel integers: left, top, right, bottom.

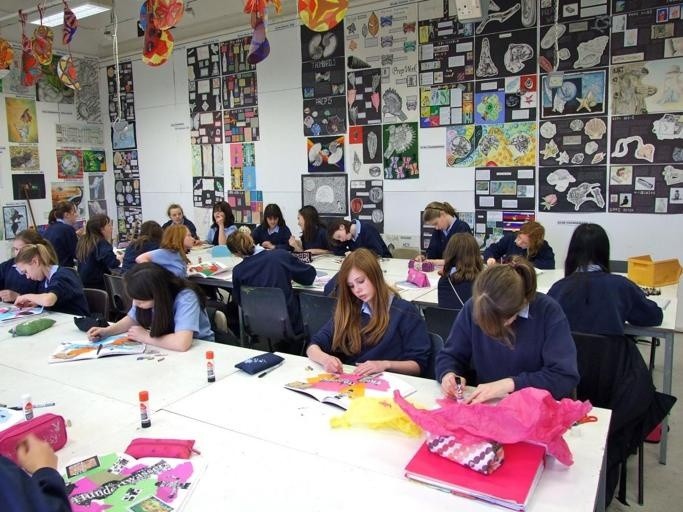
31;402;56;408
258;364;282;377
97;345;102;354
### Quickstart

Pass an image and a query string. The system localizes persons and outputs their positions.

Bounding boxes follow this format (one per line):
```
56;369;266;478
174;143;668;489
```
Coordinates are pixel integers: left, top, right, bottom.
122;220;163;278
135;222;227;331
249;204;295;252
87;262;215;350
75;214;123;287
164;203;197;244
0;229;59;304
483;221;556;269
44;199;79;270
548;224;663;427
327;218;393;258
0;433;73;512
227;232;318;348
15;242;90;316
435;256;622;511
288;206;335;258
422;203;473;260
438;231;496;310
304;250;432;377
207;202;237;248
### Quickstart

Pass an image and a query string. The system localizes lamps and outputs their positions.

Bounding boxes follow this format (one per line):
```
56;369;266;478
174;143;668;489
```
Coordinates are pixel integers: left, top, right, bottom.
29;0;113;28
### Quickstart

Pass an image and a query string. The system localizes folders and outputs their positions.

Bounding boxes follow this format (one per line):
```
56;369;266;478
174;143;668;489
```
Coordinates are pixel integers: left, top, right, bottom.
405;435;547;511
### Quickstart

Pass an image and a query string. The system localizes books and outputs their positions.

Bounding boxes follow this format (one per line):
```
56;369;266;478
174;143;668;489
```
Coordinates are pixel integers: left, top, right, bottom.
46;333;145;364
284;369;410;412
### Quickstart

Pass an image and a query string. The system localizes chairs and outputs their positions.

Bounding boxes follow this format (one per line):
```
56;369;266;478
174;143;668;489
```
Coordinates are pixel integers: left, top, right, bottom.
300;293;338;356
205;307;227;344
571;331;644;507
83;287;110;322
426;332;444;380
391;248;419;258
103;274;133;321
237;286;303;353
609;260;660;370
423;306;461;345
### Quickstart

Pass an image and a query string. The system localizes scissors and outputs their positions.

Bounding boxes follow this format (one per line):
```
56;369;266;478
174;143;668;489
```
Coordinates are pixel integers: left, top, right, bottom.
572;414;597;426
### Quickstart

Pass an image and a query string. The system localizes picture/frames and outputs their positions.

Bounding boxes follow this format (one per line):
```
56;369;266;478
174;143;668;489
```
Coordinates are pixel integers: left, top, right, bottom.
3;205;28;240
301;174;348;216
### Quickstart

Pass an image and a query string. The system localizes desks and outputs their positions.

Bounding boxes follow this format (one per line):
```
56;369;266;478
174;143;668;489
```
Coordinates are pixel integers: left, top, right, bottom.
113;244;678;465
0;301;612;512
